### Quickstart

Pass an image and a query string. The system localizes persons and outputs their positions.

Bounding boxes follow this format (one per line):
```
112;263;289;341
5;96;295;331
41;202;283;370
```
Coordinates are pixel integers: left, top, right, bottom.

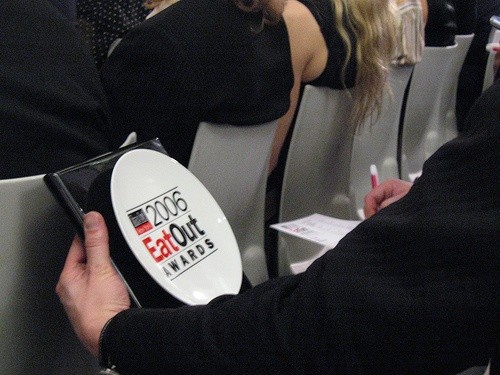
1;0;500;275
55;44;500;375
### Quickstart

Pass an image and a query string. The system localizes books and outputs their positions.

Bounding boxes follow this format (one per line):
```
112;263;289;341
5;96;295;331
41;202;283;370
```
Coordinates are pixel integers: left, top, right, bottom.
44;137;254;310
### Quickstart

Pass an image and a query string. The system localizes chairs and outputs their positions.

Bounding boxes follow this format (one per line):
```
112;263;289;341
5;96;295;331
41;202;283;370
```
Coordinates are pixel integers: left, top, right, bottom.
278;60;414;278
187;118;282;290
401;34;475;182
0;132;142;375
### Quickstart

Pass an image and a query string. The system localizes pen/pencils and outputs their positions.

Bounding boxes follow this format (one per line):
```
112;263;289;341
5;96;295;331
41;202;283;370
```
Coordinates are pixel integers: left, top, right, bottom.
370;164;381;212
